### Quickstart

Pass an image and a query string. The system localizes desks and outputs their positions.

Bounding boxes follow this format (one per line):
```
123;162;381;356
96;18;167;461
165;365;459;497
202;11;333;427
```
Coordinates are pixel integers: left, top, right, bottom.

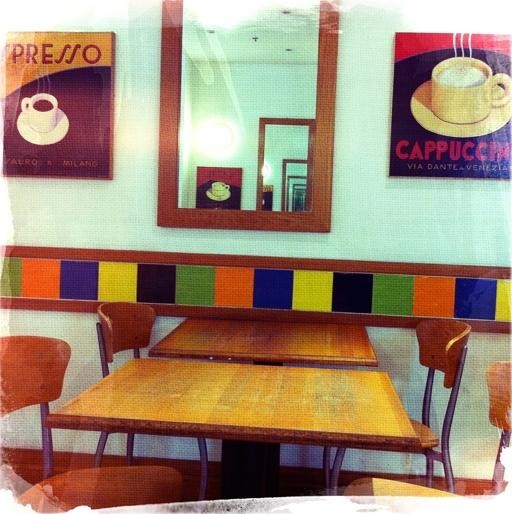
147;314;380;371
42;353;422;499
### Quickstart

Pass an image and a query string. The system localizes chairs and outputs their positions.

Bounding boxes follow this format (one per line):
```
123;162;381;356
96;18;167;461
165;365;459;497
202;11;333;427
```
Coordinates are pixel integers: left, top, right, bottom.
91;300;211;499
330;317;474;498
341;355;512;512
1;332;184;514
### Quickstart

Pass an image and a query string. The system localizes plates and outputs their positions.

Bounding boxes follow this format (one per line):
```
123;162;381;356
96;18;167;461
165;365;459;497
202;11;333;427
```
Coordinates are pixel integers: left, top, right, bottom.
205;189;231;202
410;78;511;140
17;109;69;146
430;57;512;122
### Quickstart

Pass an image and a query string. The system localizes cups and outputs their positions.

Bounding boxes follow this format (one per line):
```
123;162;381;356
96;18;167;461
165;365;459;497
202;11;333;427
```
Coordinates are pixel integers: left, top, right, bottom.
21;92;58;132
211;182;230;195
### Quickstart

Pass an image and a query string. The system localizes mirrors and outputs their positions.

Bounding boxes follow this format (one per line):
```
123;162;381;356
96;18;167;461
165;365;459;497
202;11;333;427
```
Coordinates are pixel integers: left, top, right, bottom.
156;0;342;233
